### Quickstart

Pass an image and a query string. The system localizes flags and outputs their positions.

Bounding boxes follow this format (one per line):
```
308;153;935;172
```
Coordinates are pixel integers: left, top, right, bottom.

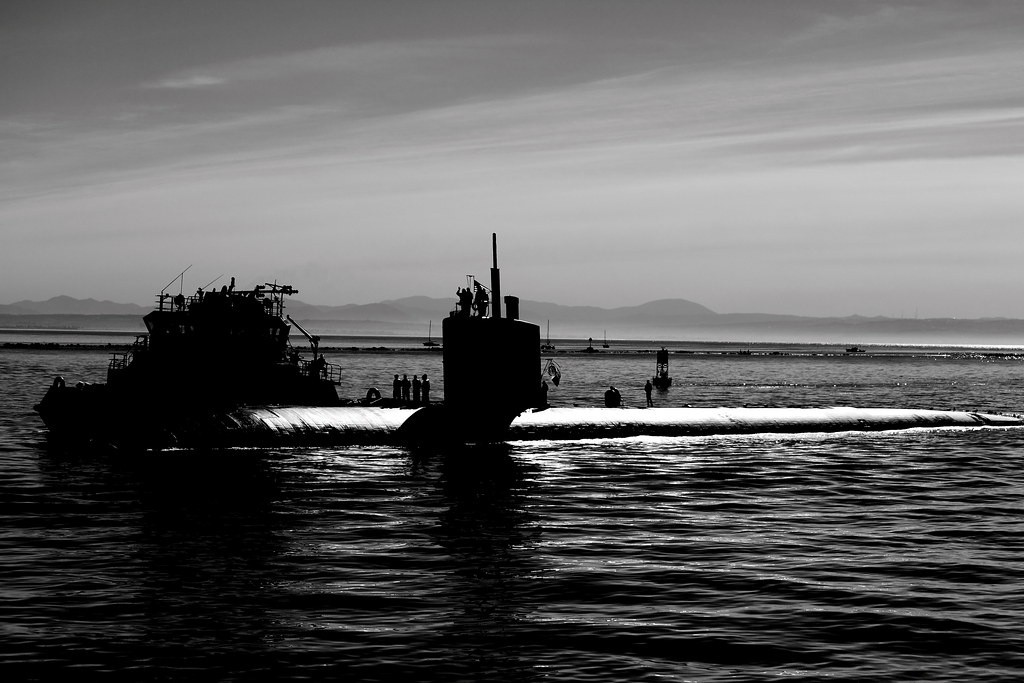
473;279;490;295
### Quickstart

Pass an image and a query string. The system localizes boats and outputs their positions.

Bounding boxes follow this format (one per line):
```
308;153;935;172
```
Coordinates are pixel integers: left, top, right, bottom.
37;284;341;456
653;346;672;388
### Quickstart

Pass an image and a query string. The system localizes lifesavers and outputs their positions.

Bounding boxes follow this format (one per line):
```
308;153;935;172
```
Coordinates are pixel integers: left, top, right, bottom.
52;376;65;388
365;386;380;400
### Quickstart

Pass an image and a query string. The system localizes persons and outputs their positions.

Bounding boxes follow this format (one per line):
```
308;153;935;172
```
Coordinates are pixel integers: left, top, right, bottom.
172;285;656;413
945;405;1022;420
